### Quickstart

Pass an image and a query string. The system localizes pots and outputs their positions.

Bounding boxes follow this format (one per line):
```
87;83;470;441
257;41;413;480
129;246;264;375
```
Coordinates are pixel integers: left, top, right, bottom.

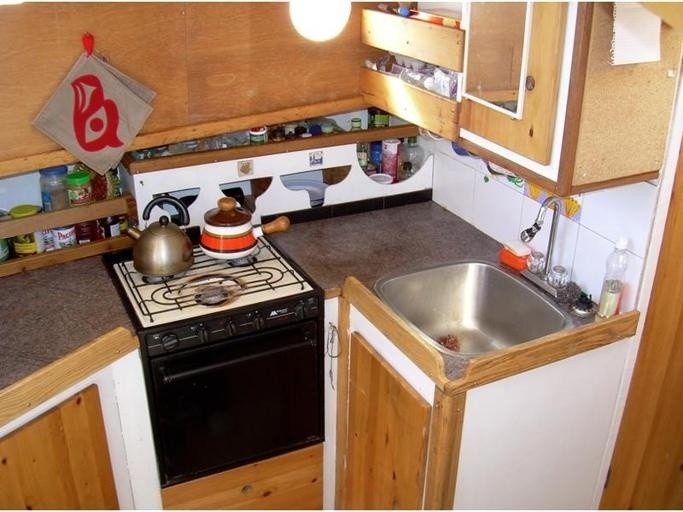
199;197;290;260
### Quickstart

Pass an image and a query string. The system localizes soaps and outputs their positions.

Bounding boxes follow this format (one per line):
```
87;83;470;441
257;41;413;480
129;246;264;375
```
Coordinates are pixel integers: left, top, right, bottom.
504;240;530;257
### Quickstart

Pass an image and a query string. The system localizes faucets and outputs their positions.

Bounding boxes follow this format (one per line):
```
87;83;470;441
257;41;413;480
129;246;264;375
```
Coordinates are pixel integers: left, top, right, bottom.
519;196;569;298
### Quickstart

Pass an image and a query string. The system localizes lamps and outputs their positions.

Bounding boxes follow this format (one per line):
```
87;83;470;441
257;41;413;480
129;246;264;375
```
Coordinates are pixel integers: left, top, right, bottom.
288;1;352;41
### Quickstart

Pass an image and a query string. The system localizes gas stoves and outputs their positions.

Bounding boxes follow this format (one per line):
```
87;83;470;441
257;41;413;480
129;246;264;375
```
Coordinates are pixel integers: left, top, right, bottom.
102;238;323;332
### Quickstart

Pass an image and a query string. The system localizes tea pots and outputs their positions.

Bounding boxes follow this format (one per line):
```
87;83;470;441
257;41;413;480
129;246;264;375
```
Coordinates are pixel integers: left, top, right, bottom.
126;197;193;277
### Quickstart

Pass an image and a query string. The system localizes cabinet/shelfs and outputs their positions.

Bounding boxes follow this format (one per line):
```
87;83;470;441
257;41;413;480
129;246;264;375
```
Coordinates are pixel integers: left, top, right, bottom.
1;258;164;511
339;280;640;510
359;3;683;196
0;3;434;269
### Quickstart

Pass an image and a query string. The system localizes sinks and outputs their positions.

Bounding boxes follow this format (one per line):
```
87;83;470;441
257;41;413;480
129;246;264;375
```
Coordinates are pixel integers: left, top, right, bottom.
374;259;572;357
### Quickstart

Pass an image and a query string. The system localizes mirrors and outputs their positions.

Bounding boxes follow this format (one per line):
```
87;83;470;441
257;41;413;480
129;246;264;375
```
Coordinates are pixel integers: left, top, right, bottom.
465;3;528;111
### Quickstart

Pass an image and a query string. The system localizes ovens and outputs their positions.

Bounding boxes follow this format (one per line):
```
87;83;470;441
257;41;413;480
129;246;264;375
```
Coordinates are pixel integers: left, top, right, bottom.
140;289;327;487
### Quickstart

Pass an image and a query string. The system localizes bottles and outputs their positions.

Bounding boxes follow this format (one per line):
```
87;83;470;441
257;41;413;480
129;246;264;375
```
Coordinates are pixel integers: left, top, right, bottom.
40;165;69;212
45;225;77;253
397;136;423;180
93;169;121;201
371;142;382;173
400;69;433;89
368;106;389;130
320;119;335;136
118;214;128;233
99;217;120;238
381;139;401;183
351;118;368;175
66;171;92;207
76;221;97;244
0;209;11;263
10;206;45;257
272;124;321;142
